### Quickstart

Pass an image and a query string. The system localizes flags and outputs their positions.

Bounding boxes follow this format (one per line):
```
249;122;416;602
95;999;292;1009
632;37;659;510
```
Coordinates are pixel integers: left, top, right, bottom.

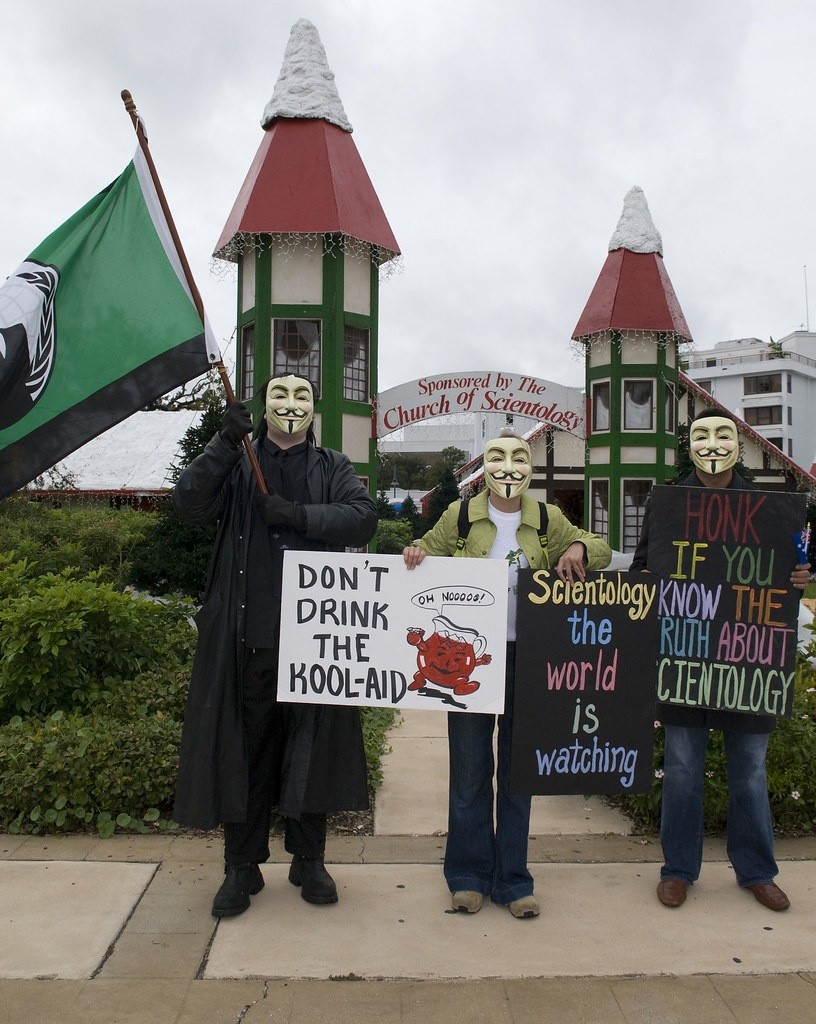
0;117;221;501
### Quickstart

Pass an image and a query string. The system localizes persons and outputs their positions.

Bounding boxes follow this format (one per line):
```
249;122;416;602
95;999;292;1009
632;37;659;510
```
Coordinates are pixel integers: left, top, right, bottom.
402;430;612;919
629;408;810;911
174;371;378;917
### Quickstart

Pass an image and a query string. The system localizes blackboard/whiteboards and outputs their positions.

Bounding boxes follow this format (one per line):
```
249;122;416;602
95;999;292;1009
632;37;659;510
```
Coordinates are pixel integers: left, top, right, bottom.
511;567;657;798
649;483;810;717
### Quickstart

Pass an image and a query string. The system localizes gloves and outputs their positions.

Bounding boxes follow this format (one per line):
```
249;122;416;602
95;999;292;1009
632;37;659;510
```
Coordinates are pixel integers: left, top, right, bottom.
255;478;305;537
219;402;253;447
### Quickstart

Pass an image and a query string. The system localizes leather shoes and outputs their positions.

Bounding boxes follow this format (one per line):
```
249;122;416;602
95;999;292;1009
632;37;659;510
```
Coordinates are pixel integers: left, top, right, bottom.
288;853;338;904
657;879;687;907
745;881;790;911
211;864;265;918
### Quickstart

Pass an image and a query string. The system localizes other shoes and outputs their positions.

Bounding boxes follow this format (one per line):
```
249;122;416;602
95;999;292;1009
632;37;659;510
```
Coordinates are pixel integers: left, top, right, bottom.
509;895;539;918
452;890;484;913
